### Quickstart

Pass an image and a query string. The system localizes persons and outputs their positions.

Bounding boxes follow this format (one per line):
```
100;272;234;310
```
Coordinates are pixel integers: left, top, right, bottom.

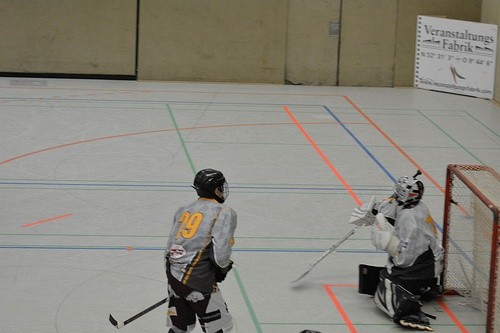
349;176;444;331
164;169;238;333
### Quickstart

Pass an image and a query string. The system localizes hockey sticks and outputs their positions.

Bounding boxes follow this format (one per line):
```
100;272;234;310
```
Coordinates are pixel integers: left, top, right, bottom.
109;297;168;330
290;170;422;284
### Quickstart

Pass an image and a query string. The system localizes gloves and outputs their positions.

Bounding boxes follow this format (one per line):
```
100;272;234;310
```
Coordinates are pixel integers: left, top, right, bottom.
215;271;227;282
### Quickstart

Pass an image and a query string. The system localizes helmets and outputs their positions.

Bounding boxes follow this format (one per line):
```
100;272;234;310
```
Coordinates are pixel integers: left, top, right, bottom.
394;176;424;208
192;168;229;204
